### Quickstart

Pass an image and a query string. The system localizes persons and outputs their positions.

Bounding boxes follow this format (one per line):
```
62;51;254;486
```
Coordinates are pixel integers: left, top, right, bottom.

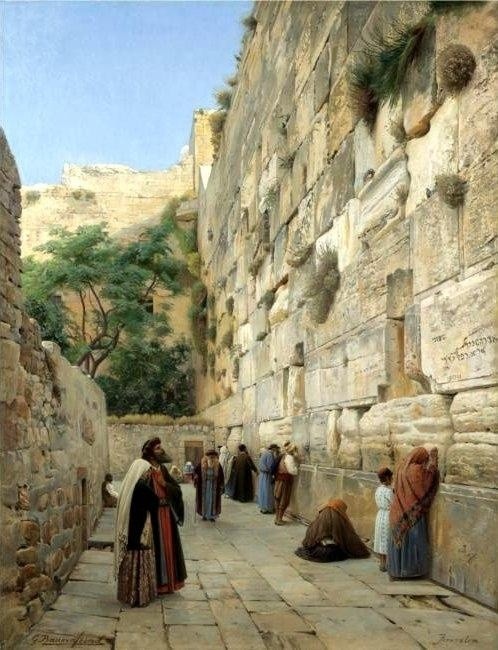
217;441;301;524
387;446;440;580
118;437;188;608
375;466;397;572
295;497;370;563
193;449;227;522
101;473;119;508
170;465;182;483
182;461;193;484
